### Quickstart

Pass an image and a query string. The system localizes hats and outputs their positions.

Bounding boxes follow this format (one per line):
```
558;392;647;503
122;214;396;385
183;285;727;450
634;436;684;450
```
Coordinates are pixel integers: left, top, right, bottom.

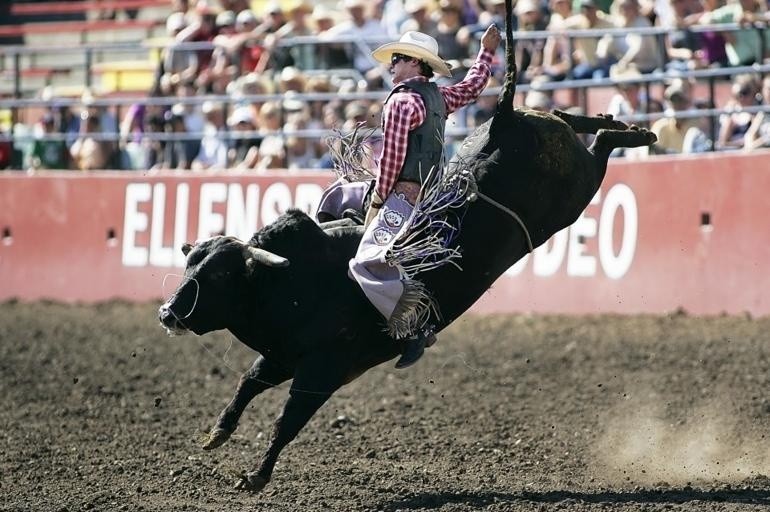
372;31;452;78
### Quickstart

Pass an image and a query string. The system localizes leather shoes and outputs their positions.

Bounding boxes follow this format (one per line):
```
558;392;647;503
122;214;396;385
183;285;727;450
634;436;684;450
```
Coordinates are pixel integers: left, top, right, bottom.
395;329;436;369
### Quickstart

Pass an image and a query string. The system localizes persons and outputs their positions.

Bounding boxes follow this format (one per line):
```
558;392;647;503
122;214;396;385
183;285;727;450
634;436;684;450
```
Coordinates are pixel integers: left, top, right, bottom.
1;0;770;171
315;23;500;370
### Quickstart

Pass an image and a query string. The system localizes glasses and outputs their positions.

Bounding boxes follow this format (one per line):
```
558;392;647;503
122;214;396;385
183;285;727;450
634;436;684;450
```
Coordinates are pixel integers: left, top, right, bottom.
392;55;404;64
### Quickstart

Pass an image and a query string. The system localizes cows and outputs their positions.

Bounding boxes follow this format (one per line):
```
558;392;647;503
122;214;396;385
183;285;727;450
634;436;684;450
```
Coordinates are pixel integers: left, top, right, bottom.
157;0;657;491
